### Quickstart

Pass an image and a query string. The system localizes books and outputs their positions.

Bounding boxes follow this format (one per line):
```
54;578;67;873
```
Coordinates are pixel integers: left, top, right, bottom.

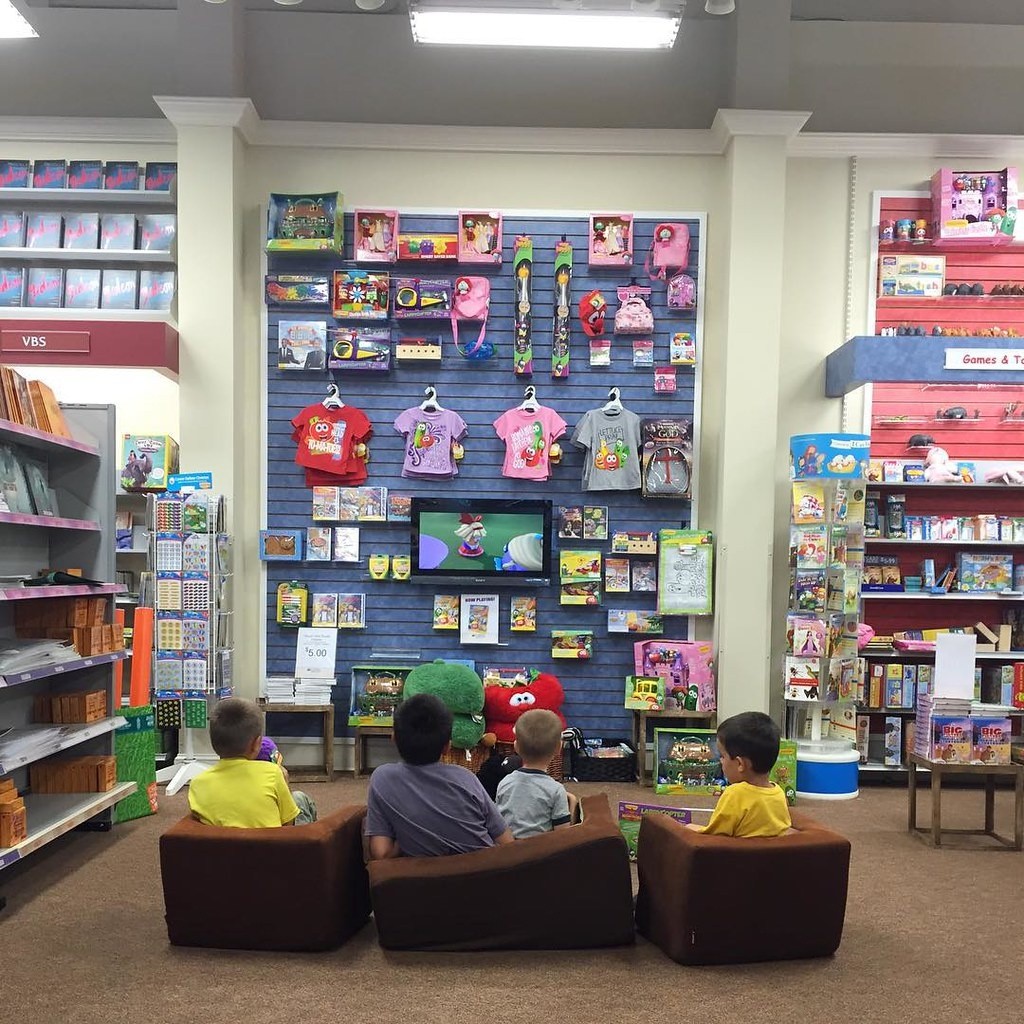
264;627;338;706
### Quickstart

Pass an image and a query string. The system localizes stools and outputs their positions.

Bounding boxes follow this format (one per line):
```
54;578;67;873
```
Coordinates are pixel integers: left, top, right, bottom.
252;697;336;785
353;726;397;781
628;709;717;787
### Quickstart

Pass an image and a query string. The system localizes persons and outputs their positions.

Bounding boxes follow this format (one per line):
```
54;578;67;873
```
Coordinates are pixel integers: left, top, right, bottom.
494;708;577;842
365;694;516;862
684;712;792;836
186;698;319;828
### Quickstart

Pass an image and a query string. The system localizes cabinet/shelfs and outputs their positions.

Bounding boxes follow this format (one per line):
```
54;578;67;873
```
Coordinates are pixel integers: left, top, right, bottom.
116;464;154;702
0;180;180;322
0;399;141;885
857;416;1024;792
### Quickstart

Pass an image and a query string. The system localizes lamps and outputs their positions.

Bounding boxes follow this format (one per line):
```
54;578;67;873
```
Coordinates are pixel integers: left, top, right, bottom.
0;0;45;41
407;0;691;54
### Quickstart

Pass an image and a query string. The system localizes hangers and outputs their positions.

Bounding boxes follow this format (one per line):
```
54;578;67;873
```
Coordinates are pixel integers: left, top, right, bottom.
518;383;540;413
321;382;345;410
604;387;623;413
421;384;442;413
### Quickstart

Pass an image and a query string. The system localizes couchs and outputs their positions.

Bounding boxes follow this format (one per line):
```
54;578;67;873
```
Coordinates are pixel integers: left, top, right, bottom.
367;790;642;954
154;801;368;956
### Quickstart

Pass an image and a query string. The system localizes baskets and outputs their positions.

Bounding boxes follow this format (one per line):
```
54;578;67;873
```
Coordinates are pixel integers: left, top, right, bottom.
441;745;491;776
492;738;564;783
569;736;638;783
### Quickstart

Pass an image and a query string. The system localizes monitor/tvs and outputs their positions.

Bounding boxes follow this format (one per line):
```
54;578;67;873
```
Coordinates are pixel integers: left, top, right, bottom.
410;497;552;586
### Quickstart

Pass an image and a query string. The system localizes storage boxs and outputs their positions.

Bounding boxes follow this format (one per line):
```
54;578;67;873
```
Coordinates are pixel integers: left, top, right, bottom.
0;158;177;308
875;253;948;299
930;166;1020;245
856;459;1024;769
615;798;691;863
247;184;697;397
30;755;118;794
15;601;126;656
768;738;799;809
569;736;637;785
32;687;107;726
167;469;216;493
258;482;716;728
122;432;179;496
652;724;731;798
0;776;27;849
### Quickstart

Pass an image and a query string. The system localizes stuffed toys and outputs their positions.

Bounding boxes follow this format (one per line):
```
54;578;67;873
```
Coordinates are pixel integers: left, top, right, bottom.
402;659;486;752
486;672;567;742
922;446;961;484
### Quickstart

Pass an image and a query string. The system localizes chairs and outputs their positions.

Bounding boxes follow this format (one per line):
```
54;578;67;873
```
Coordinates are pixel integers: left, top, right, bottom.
633;812;853;974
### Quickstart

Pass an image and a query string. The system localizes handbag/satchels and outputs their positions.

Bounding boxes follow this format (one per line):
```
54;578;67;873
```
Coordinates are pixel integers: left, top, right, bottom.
613;297;654;335
645;223;691;282
450;276;490;357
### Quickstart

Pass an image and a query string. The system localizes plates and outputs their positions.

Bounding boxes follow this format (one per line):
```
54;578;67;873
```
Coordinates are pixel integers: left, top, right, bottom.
420;533;449;569
